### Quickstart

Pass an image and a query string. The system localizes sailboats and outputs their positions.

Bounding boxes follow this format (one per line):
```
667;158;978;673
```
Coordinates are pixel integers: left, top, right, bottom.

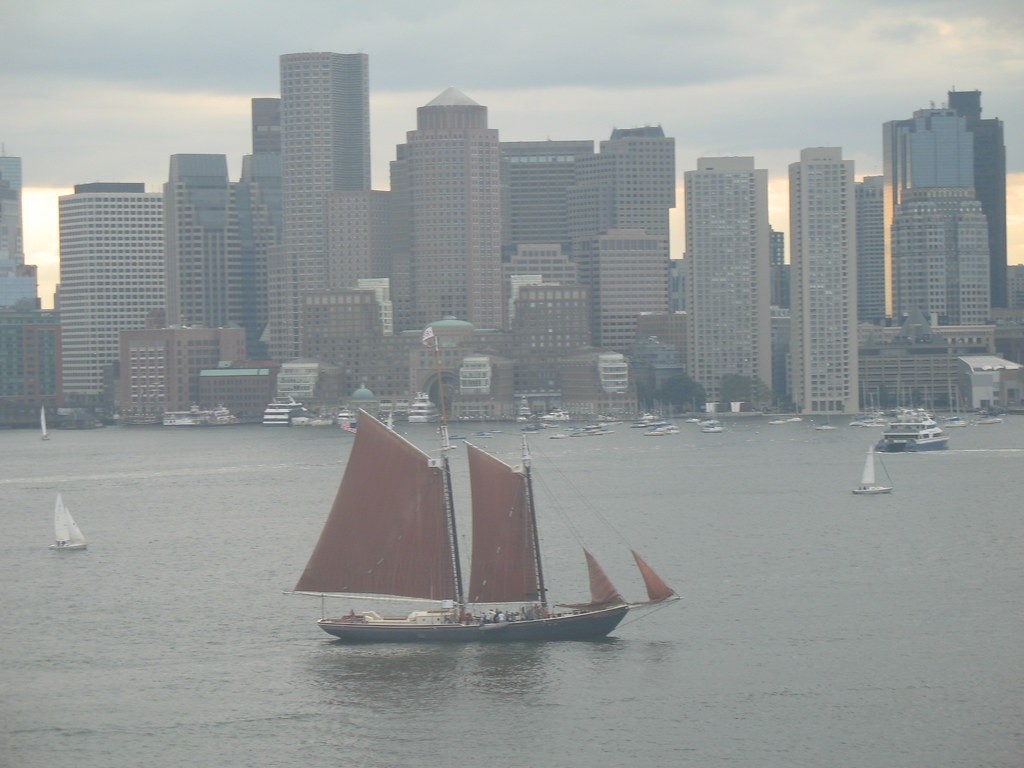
47;491;87;551
848;443;896;497
41;404;49;442
278;409;682;640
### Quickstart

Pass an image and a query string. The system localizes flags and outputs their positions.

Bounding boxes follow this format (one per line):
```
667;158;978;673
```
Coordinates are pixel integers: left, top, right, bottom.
423;327;434;340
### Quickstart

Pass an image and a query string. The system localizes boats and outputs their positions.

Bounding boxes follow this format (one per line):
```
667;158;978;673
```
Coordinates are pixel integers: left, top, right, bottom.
425;408;1011;463
408;391;439;422
